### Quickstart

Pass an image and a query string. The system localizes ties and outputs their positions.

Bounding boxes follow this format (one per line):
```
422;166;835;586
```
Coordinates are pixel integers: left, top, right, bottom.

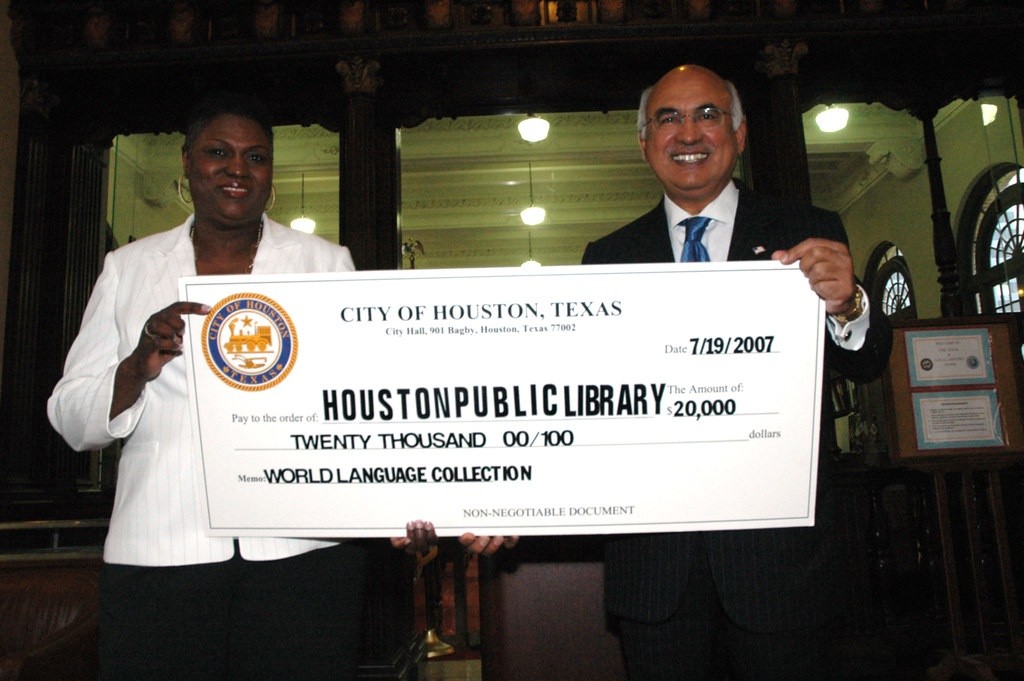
678;216;712;263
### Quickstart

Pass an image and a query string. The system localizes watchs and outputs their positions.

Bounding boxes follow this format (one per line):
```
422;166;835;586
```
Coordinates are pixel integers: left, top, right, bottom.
832;286;865;323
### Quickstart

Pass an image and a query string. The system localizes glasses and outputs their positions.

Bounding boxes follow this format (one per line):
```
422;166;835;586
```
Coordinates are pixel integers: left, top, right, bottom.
643;107;734;131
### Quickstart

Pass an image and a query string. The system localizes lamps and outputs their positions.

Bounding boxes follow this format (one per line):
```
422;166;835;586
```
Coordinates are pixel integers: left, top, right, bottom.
517;113;551;142
290;172;317;234
814;104;849;132
520;162;545;226
520;232;540;268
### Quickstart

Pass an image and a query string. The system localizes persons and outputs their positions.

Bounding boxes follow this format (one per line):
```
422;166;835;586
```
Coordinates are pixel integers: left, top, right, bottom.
45;92;441;681
458;64;894;681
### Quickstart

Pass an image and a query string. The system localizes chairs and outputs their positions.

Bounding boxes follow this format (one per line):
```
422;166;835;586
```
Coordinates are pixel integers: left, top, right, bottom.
0;568;100;681
